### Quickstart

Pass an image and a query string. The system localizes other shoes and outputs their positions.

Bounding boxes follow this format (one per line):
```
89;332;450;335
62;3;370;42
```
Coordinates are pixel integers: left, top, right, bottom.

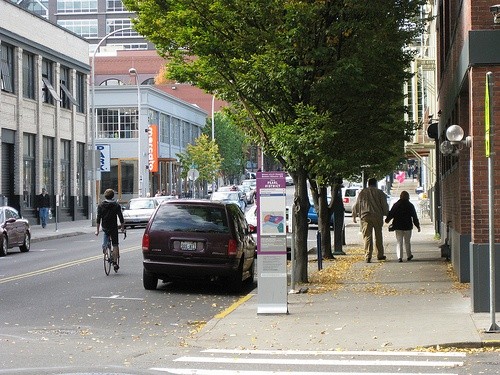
377;255;386;260
398;258;403;262
366;258;370;262
407;255;413;260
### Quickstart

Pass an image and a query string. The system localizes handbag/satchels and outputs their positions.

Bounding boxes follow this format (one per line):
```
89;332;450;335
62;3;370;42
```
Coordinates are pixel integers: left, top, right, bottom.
388;224;394;231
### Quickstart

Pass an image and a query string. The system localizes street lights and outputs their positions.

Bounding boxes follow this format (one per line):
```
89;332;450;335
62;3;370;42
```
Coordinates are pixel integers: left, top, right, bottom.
128;67;143;199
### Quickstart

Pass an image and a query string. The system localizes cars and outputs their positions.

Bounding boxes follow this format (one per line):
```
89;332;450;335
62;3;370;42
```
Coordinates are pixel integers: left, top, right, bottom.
122;198;157;229
0;206;32;256
209;172;362;232
141;198;258;293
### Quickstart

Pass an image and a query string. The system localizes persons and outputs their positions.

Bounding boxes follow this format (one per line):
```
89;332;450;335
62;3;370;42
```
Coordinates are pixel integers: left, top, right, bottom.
154;190;162;197
36;187;52;229
95;189;126;271
161;189;166;196
385;191;421;263
257;168;261;172
170;189;177;196
396;159;420;179
351;178;390;263
